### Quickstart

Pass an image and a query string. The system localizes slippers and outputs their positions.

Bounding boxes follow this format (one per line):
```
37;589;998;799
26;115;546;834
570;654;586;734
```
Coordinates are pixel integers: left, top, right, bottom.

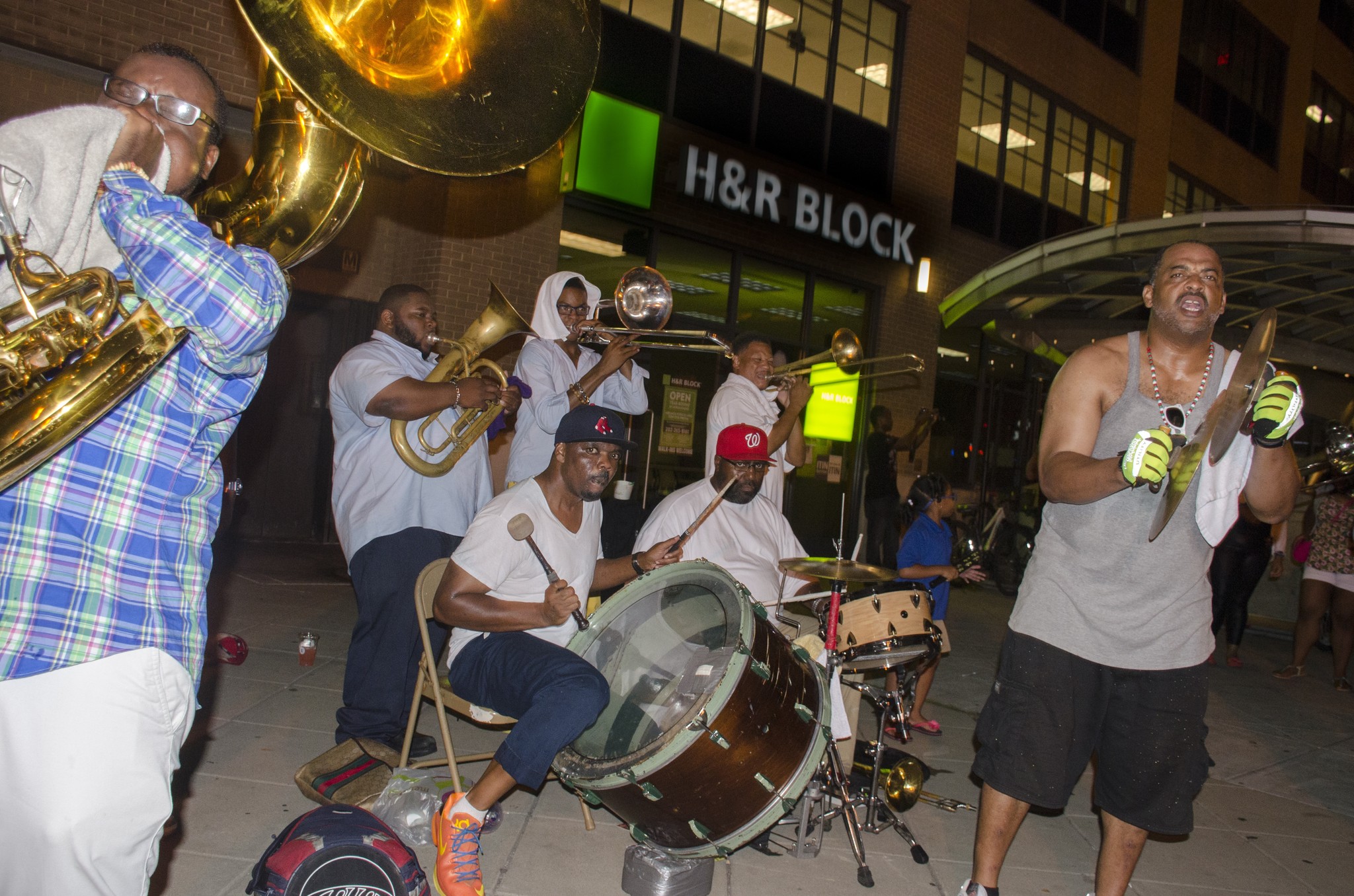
882;726;913;741
911;720;943;736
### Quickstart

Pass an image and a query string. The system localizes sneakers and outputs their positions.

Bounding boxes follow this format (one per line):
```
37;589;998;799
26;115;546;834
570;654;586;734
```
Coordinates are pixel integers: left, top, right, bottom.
430;791;486;896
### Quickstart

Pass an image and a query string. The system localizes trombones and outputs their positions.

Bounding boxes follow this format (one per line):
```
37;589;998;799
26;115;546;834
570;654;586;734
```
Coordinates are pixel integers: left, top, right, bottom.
760;326;928;393
563;265;733;357
851;755;979;813
1297;419;1353;491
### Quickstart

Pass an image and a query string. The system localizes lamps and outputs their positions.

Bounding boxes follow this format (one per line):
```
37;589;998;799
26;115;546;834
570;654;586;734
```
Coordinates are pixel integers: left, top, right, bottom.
915;252;933;297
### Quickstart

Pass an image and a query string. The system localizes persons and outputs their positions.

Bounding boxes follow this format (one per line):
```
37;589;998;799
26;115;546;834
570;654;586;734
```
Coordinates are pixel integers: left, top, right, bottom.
866;404;939;590
502;271;649;493
329;284;522;758
957;242;1302;896
1207;475;1354;691
883;473;986;739
706;332;815;514
432;404;690;896
0;42;290;896
632;423;883;809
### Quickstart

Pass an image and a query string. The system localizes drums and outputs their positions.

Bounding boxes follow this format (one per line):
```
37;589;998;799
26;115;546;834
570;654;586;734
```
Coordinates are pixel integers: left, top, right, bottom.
549;557;833;860
818;580;941;674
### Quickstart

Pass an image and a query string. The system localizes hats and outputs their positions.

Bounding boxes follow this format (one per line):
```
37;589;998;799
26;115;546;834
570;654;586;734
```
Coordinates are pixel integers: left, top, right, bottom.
554;404;637;450
716;423;777;463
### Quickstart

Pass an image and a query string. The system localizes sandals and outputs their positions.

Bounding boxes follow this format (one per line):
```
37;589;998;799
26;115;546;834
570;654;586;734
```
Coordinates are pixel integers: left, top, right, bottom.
1273;664;1306;679
1329;672;1354;691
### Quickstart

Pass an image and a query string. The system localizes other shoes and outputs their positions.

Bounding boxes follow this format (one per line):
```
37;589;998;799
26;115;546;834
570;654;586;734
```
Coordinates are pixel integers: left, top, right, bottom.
958;879;989;896
1207;655;1217;664
1225;657;1243;668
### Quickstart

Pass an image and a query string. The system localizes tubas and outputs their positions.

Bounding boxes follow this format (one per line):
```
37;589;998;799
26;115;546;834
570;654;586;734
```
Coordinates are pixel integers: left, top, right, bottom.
389;276;543;478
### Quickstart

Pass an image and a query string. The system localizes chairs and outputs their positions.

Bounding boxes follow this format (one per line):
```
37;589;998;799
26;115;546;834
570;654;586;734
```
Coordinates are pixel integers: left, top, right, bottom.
400;558;595;830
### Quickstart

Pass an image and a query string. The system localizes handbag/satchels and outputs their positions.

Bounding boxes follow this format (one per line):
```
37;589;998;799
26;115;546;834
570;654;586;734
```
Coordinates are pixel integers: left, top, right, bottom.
375;768;475;855
1292;537;1314;563
293;739;428;815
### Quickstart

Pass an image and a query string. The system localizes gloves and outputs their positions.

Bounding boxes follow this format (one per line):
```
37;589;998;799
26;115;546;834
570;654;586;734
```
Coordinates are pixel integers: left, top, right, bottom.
1118;427;1187;489
1242;374;1303;448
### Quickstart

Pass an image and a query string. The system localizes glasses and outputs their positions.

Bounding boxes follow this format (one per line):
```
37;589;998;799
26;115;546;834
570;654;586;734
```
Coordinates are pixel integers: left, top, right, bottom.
719;455;771;473
555;303;591;316
103;73;223;134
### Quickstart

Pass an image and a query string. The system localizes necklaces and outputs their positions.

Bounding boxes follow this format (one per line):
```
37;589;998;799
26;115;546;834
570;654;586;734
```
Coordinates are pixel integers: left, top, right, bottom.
1146;331;1214;430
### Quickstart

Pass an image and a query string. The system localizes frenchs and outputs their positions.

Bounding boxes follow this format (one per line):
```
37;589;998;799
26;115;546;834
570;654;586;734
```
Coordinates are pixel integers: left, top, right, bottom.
0;0;599;488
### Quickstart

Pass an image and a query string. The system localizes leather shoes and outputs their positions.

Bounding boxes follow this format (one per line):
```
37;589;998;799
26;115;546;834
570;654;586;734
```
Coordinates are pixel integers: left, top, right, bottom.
336;724;438;757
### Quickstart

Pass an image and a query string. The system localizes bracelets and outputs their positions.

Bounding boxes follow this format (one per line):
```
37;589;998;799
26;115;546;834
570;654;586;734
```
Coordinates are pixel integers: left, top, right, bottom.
632;550;646;575
97;161;150;200
569;384;586;405
575;382;590;404
449;381;460;409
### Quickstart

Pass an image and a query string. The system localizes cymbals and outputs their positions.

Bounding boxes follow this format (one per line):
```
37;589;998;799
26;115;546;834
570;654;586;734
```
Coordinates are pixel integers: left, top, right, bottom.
1147;387;1229;541
1206;305;1277;468
777;557;900;582
